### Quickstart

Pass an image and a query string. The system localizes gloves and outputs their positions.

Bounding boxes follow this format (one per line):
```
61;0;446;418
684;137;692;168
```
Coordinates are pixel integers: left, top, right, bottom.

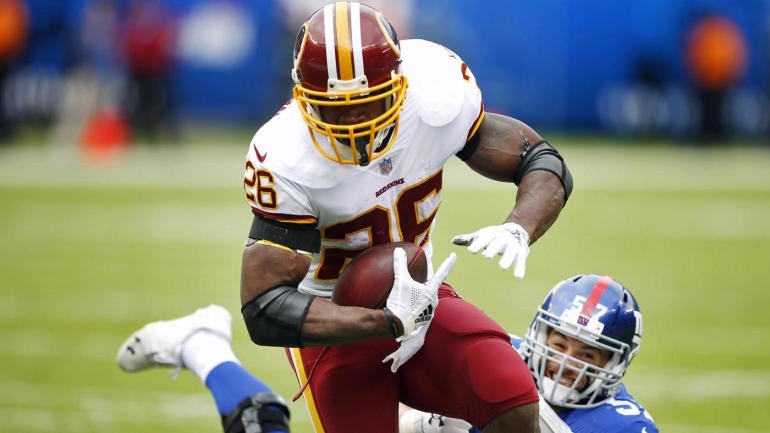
448;221;532;277
387;244;460;338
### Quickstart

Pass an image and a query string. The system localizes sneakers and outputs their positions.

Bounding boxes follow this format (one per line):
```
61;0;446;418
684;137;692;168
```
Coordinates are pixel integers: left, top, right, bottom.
113;302;234;374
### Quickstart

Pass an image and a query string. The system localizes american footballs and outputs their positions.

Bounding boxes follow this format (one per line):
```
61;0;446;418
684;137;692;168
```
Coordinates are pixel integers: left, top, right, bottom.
332;241;429;310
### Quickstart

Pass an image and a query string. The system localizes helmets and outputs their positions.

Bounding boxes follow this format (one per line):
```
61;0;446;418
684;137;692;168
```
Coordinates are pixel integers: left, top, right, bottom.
520;275;643;409
287;2;404;164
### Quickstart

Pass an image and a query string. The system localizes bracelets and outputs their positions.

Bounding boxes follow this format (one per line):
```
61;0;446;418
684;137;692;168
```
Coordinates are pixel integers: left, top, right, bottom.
384;307;400;342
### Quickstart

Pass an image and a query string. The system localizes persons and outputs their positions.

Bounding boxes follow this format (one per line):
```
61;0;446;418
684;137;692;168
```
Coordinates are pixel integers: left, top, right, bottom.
240;0;572;433
114;273;657;433
